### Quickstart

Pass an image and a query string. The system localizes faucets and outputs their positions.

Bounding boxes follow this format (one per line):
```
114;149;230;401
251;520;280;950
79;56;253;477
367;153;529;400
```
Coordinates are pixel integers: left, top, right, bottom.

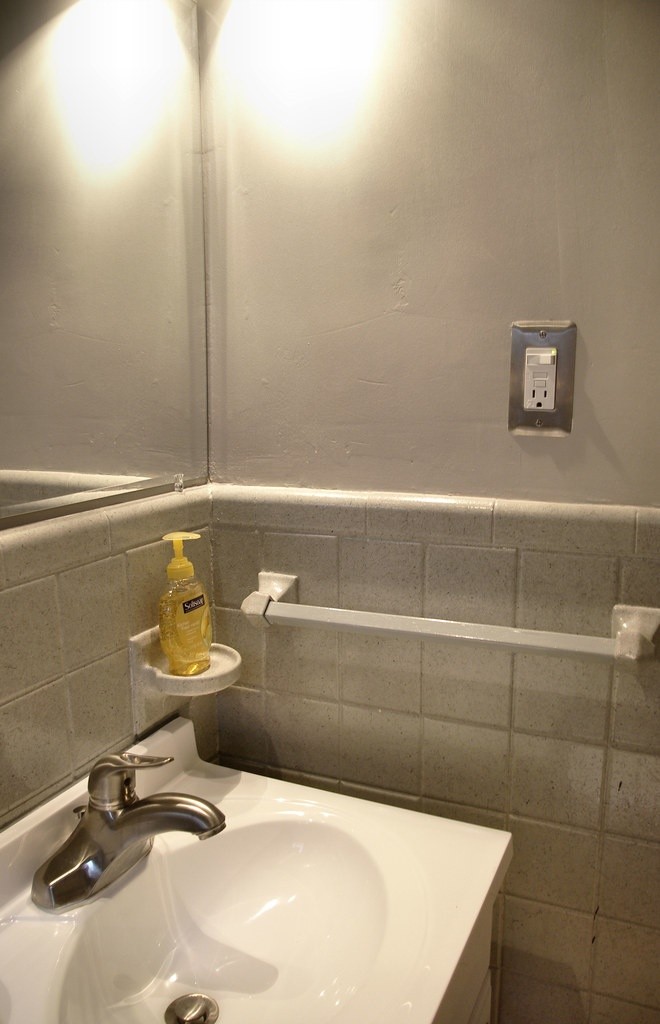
34;751;228;917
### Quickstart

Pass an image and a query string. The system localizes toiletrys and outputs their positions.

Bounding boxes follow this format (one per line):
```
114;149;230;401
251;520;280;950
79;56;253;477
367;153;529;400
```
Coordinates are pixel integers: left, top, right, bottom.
157;533;214;676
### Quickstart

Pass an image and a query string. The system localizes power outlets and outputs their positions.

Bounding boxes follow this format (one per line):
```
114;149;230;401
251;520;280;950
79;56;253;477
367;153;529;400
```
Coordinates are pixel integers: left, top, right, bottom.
508;320;577;437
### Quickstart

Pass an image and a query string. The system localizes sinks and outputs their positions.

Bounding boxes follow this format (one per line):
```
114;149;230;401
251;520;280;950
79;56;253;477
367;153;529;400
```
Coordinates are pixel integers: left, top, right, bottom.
58;817;388;1024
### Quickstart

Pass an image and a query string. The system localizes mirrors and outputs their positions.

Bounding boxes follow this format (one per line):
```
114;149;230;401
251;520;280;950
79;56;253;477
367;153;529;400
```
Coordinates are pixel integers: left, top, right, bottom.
0;0;211;531
